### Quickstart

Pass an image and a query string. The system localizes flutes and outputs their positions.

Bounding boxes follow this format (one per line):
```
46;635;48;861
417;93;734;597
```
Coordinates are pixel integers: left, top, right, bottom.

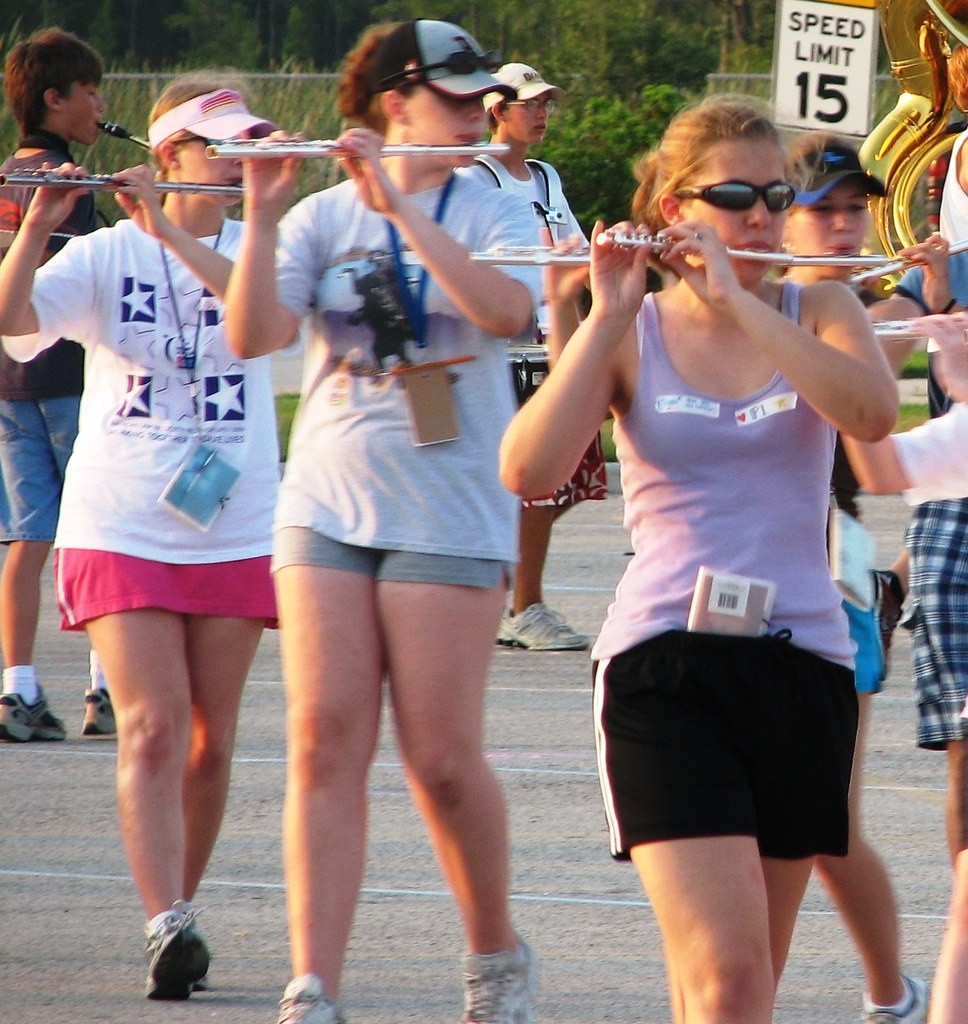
873;313;968;342
468;241;896;267
847;239;968;285
595;228;795;263
206;137;511;161
0;168;245;198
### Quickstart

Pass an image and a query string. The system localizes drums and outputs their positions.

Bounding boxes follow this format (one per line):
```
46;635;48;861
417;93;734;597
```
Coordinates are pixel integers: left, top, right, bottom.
505;344;553;411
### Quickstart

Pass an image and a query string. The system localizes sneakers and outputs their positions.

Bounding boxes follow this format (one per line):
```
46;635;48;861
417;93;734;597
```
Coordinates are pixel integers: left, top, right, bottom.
861;969;929;1024
81;688;116;734
0;684;66;743
275;974;344;1024
867;570;904;681
144;899;211;1001
495;603;591;650
459;938;537;1024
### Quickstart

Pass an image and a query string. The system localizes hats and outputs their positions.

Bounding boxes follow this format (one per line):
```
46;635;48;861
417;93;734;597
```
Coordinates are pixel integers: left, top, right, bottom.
367;19;518;101
483;62;565;112
794;147;886;206
148;88;278;150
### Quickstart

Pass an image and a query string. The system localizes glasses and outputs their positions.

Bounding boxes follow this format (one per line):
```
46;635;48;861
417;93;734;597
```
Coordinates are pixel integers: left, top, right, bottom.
377;50;505;84
673;180;796;212
507;99;558;112
172;136;223;147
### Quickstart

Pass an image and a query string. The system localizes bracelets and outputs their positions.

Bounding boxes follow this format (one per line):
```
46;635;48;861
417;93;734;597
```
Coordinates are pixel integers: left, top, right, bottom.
945;298;955;313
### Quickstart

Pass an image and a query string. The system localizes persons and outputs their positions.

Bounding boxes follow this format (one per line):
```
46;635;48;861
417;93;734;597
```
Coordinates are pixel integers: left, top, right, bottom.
0;30;117;742
498;91;899;1023
840;312;968;507
468;63;589;651
852;125;967;1024
545;135;928;1024
225;17;545;1024
0;73;280;999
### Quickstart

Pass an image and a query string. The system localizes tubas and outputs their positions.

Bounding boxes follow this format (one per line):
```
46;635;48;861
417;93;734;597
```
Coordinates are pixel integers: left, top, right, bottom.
857;0;968;297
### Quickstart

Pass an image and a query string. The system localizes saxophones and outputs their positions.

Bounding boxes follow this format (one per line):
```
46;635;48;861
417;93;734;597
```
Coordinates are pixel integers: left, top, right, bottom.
97;120;153;151
